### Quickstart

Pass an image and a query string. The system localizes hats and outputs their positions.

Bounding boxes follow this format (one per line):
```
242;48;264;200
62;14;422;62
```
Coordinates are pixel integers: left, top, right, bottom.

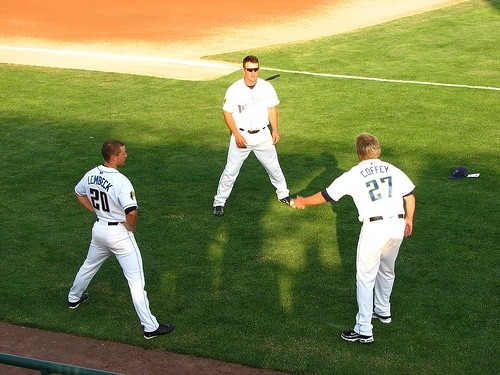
448;166;469;180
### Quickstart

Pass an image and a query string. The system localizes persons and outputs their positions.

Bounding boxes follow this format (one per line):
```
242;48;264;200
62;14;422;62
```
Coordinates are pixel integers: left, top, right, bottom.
214;57;293;215
290;133;416;343
68;141;175;339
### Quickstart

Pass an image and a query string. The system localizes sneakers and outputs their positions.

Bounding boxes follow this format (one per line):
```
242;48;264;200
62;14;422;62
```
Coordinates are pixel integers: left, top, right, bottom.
143;322;174;340
68;292;90;309
213;206;225;217
278;196;290;205
372;311;392;324
341;329;375;344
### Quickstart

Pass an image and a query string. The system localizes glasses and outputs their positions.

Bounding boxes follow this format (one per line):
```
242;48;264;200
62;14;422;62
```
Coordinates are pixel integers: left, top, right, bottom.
245;67;259;72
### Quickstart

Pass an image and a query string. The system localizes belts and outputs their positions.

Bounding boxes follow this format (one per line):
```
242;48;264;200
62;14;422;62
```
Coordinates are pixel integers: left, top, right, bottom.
238;124;268;134
370;213;405;222
96;218;118;225
